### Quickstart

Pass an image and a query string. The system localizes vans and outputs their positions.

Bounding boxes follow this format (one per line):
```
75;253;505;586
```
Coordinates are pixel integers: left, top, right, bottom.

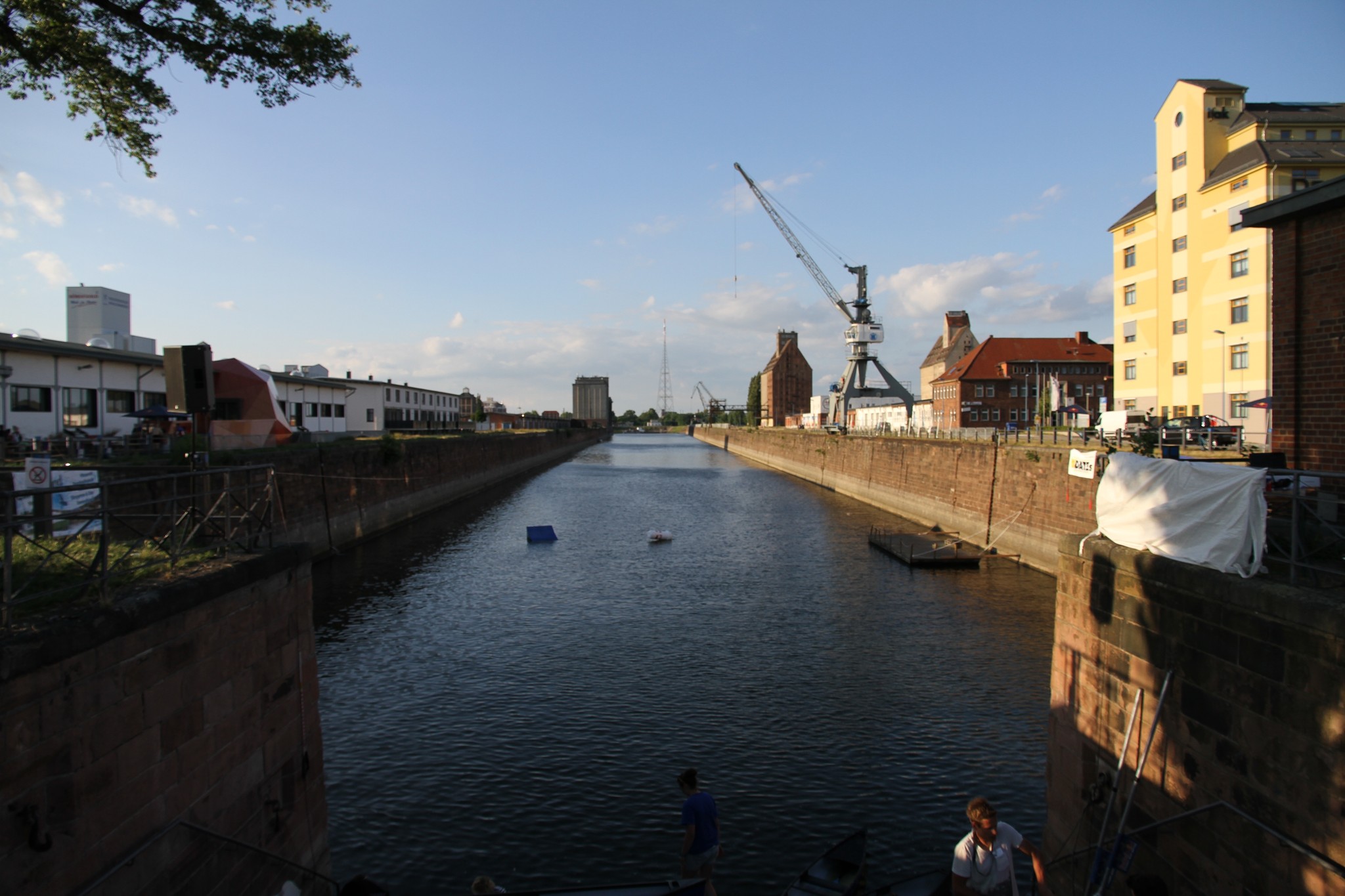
1093;410;1150;443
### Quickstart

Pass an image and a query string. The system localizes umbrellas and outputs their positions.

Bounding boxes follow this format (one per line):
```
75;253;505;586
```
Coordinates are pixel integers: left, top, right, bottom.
1056;404;1090;440
120;400;192;425
1237;396;1272;453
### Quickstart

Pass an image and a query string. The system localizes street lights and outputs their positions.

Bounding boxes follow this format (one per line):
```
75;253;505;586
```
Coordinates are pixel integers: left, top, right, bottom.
1029;359;1039;436
1025;373;1029;429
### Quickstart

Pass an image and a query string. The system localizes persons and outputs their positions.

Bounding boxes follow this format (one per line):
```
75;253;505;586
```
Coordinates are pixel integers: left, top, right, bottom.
0;424;24;454
131;423;186;448
949;795;1054;896
677;767;721;894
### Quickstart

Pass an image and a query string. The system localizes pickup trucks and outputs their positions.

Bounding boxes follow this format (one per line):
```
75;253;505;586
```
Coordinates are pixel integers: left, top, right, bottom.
1129;416;1246;450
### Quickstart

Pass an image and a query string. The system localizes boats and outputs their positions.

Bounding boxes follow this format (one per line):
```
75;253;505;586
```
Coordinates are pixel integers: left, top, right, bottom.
780;825;871;896
337;867;710;896
860;868;949;896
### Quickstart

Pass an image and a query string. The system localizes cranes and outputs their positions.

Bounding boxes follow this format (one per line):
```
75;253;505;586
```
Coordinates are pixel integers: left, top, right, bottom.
732;162;917;437
